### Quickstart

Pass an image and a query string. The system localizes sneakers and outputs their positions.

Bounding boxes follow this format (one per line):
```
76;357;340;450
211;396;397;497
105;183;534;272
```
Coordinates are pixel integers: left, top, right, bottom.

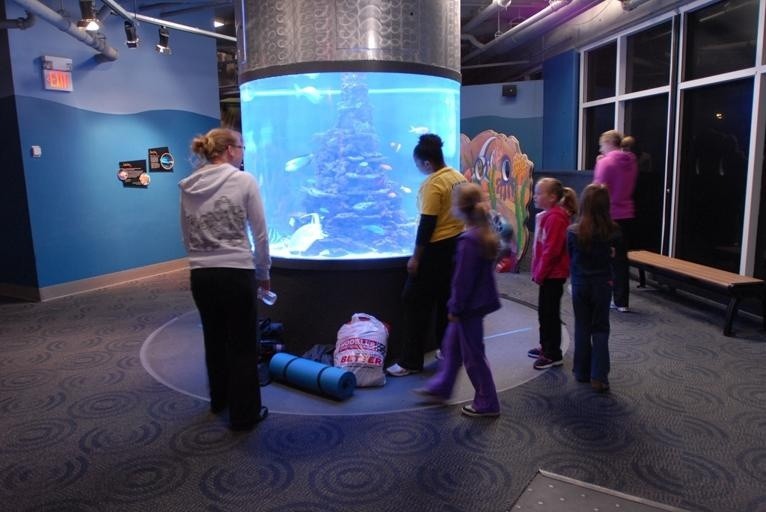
386;363;421;377
434;348;463;366
461;403;501;418
611;294;631;312
410;387;449;404
533;356;564;370
591;379;610;391
526;344;545;358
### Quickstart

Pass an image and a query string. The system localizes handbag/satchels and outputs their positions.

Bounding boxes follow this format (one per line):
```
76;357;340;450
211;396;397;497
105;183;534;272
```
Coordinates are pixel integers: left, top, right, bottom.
301;343;336;367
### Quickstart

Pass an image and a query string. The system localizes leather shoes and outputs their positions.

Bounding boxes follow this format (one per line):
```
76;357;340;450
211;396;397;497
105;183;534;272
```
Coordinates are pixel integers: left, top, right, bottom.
257;406;268;423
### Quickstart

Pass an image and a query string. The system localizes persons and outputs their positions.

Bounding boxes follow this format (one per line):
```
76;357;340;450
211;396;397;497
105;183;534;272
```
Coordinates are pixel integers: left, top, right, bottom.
386;134;467;376
568;183;622;392
410;182;502;418
527;177;577;370
176;128;272;432
592;130;638;311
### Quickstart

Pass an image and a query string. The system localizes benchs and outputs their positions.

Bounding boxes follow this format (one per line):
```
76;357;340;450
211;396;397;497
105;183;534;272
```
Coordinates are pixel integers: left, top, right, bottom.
627;248;766;338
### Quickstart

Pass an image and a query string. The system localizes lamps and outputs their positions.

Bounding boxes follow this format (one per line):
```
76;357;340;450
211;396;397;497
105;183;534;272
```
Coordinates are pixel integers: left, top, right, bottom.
75;1;173;58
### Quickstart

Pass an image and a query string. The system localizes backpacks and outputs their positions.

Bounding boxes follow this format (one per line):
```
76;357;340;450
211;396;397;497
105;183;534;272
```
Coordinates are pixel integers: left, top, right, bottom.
253;314;288;361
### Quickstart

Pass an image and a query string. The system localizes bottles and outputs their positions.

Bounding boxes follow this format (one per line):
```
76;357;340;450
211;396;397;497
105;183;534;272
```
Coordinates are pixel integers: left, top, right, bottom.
255;346;273;386
256;286;278;306
